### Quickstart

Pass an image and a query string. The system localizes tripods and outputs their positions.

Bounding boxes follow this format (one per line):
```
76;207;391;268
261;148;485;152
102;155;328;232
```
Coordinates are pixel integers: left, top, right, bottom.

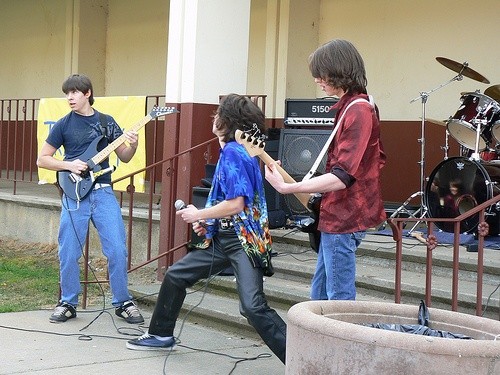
377;74;461;237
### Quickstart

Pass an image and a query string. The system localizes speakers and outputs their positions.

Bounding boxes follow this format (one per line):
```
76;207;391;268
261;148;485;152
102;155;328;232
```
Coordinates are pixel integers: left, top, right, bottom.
278;128;334;219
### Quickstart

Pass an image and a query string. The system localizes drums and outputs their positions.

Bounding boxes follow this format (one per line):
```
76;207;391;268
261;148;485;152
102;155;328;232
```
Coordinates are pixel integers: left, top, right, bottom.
446;92;500;152
491;111;500;144
425;155;500;238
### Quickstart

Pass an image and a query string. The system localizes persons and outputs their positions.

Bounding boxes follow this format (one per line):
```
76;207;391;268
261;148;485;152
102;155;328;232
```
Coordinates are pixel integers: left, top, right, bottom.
35;73;145;323
127;94;287;365
265;39;387;301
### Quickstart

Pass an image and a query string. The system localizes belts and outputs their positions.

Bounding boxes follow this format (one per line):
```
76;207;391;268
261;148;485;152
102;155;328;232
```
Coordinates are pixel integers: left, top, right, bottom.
219;218;234;230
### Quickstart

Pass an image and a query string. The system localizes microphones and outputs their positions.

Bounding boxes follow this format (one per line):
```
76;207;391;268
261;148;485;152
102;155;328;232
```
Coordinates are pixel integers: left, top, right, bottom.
175;199;206;228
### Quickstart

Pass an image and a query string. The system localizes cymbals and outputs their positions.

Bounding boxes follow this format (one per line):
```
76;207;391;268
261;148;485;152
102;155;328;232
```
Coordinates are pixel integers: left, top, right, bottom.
420;116;446;126
435;57;490;84
485;84;500;105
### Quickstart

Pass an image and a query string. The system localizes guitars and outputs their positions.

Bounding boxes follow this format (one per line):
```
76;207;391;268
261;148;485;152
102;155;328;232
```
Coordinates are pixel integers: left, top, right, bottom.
235;125;323;253
57;104;178;202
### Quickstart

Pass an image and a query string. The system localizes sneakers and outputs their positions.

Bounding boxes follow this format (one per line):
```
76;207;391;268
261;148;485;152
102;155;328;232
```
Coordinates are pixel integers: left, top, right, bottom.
115;300;144;323
126;329;177;350
49;302;76;323
